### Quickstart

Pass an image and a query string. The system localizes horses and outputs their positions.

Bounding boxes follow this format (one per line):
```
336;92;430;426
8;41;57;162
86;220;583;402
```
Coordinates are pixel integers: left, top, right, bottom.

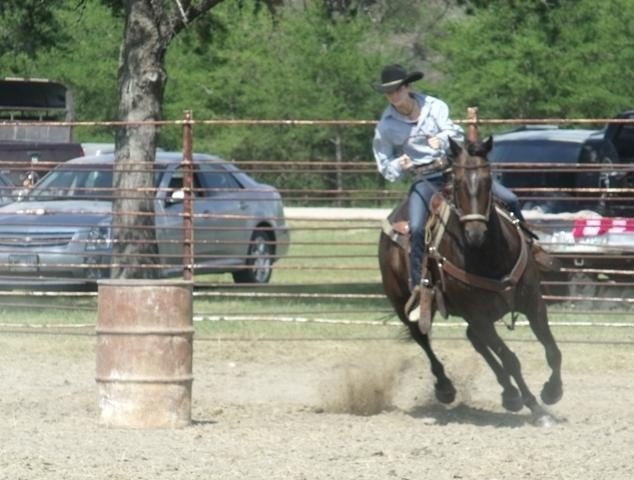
378;134;563;428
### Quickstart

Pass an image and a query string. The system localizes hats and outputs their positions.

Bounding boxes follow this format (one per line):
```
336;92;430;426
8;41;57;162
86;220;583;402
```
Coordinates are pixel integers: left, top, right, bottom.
370;64;423;94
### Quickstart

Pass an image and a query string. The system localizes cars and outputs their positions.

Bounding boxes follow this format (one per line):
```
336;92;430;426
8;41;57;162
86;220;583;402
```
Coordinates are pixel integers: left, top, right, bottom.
0;146;296;284
477;110;634;305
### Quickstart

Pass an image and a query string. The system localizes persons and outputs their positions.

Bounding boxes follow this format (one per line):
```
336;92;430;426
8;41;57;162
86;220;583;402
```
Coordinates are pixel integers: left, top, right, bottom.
17;177;34;201
368;63;525;323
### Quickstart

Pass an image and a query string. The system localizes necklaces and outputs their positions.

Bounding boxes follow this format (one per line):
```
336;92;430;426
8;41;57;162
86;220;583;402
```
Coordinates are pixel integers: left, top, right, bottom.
395;99;417;116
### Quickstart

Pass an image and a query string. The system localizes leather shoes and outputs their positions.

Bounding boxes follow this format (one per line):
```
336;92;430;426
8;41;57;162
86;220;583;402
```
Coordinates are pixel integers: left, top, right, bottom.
533;248;553;271
408;298;420;322
